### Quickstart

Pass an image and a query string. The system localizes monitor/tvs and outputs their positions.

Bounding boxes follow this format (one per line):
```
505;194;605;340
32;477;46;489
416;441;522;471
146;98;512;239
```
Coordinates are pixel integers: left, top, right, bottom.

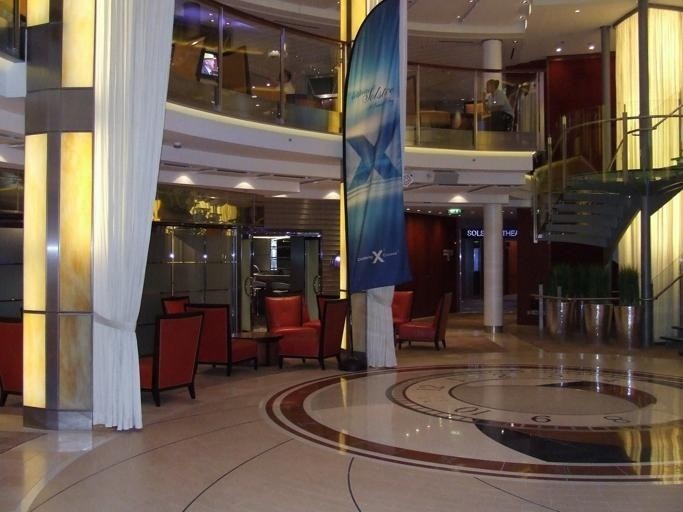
196;51;219;80
306;73;338;99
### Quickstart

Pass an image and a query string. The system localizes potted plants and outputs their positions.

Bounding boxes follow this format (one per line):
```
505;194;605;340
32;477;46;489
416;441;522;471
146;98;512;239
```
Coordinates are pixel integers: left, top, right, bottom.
580;269;612;342
614;263;642;349
545;271;578;344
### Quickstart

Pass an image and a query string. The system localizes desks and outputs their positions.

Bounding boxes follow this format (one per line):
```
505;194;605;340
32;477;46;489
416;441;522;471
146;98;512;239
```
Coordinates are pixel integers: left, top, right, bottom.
252;87;287;103
420;110;453;126
465;100;487;125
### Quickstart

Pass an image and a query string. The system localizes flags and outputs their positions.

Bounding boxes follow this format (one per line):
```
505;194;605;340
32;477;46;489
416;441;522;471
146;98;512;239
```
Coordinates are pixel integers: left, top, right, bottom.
342;0;414;295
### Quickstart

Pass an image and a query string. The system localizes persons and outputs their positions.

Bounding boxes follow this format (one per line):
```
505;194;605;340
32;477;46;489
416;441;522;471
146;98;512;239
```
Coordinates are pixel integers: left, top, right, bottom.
274;69;296;95
482;78;516;132
201;53;217;75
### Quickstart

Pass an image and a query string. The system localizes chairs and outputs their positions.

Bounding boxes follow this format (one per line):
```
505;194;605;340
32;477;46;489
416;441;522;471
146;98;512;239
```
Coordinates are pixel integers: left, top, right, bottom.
399;292;453;352
160;296;190;314
263;290;348;369
186;304;259;376
1;316;23;408
140;310;204;408
392;290;415;348
